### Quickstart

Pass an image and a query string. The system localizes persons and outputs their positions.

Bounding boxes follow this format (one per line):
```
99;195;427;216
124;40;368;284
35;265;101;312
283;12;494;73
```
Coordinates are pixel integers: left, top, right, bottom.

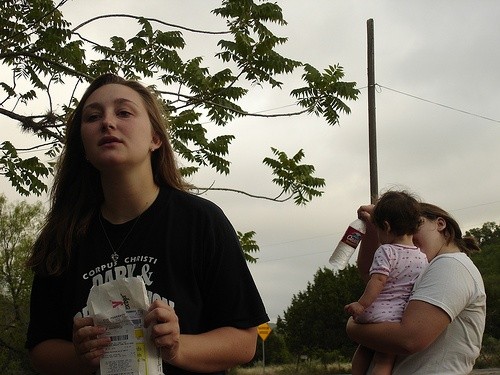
345;202;487;374
24;73;270;375
343;191;429;375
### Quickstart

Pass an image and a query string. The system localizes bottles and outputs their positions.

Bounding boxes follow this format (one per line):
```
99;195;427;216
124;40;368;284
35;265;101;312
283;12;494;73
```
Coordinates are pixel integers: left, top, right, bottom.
329;217;367;271
